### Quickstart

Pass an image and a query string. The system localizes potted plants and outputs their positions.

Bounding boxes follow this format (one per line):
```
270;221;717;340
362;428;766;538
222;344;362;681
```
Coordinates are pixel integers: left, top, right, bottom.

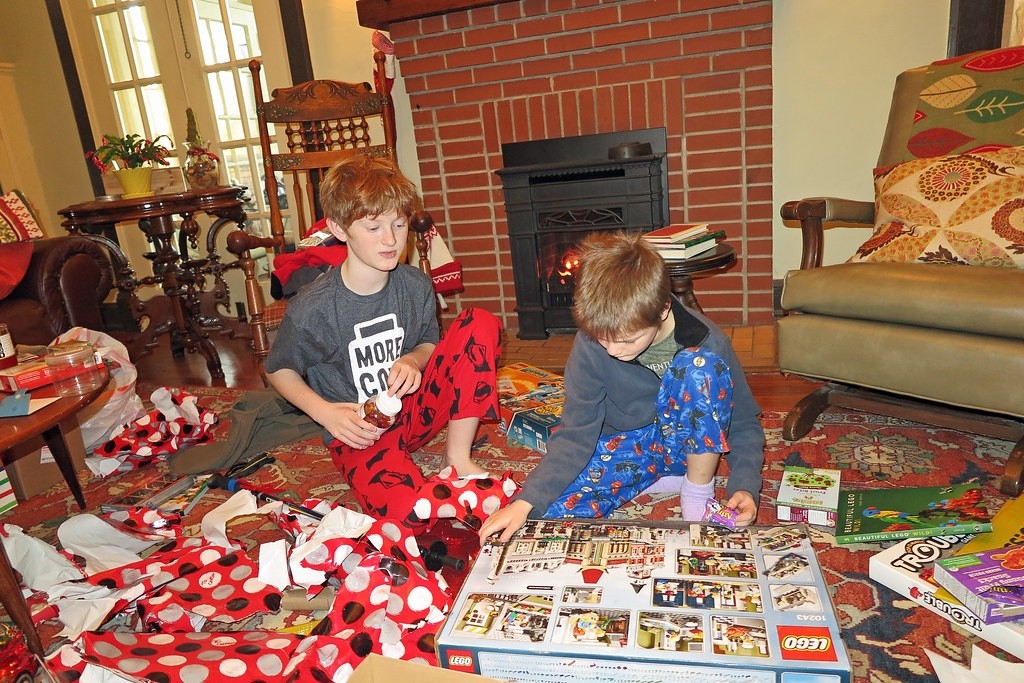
180;107;220;190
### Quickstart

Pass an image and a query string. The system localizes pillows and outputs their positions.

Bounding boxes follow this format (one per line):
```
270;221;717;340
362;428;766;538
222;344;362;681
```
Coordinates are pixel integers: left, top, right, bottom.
0;242;34;301
843;145;1024;269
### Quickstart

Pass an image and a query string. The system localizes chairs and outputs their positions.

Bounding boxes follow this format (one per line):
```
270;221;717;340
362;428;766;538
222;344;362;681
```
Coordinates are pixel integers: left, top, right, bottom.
775;45;1024;496
227;51;434;387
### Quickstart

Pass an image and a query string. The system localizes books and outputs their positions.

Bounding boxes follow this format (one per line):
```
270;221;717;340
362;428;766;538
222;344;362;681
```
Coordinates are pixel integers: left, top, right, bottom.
834;482;993;544
641;223;725;260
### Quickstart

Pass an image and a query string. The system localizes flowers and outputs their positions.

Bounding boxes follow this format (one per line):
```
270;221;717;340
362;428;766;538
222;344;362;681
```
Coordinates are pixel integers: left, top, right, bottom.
84;135;174;176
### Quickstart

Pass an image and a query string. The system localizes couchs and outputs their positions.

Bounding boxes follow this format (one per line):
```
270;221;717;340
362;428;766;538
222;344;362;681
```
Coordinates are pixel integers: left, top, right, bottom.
0;184;114;347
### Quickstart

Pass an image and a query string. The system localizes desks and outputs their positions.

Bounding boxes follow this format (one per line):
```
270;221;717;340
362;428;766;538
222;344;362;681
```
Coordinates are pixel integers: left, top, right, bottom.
57;184;253;382
0;366;112;511
662;242;737;317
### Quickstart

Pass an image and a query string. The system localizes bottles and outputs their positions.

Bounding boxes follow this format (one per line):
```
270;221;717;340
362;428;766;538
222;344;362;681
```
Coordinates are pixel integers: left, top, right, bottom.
356;390;402;438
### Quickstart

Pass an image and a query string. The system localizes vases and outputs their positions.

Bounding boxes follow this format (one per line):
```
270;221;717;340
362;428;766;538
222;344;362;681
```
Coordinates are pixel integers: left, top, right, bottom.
110;167;152;195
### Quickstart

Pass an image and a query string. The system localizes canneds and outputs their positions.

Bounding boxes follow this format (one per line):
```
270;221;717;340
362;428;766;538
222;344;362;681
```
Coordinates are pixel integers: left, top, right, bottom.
0;324;18;369
356;391;403;437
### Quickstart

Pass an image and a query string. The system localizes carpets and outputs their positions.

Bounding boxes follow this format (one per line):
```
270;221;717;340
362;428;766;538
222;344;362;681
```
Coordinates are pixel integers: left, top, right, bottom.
0;383;1024;683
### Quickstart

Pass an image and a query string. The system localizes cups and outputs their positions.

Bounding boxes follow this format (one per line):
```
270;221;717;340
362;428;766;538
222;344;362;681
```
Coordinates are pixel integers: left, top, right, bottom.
44;346;102;397
0;323;18;370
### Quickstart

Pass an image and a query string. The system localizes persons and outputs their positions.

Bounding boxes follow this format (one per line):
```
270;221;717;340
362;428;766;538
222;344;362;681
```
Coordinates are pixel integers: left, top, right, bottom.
262;154;523;525
477;225;766;548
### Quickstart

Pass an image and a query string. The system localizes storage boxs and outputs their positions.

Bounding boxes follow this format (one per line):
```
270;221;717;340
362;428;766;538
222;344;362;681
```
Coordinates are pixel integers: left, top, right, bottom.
0;352;105;393
432;517;856;683
2;412;87;502
0;457;18;515
479;362;565;455
869;533;1024;661
775;466;841;527
